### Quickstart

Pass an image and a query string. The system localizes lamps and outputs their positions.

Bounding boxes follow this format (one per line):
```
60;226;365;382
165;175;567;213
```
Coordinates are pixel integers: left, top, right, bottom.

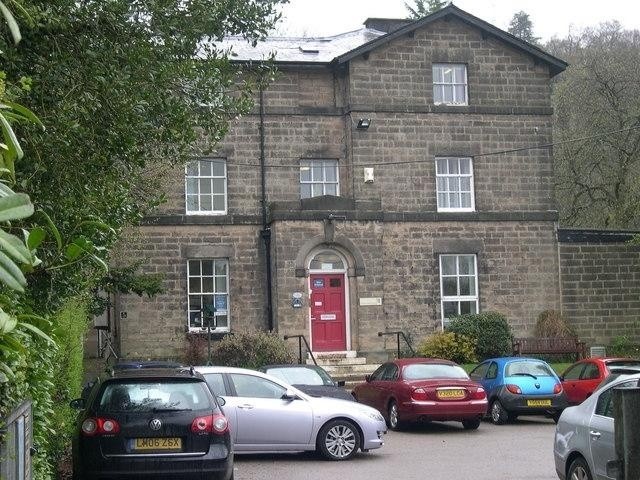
357;118;372;130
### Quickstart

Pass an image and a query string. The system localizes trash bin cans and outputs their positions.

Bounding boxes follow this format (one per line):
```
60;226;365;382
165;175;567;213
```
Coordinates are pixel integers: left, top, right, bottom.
612;387;640;480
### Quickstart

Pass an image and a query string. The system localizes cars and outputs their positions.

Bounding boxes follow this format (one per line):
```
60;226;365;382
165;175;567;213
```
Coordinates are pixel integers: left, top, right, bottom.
551;372;639;480
467;354;569;427
177;362;390;462
79;357;190;410
67;365;237;480
553;355;640;425
349;356;490;433
251;362;360;405
